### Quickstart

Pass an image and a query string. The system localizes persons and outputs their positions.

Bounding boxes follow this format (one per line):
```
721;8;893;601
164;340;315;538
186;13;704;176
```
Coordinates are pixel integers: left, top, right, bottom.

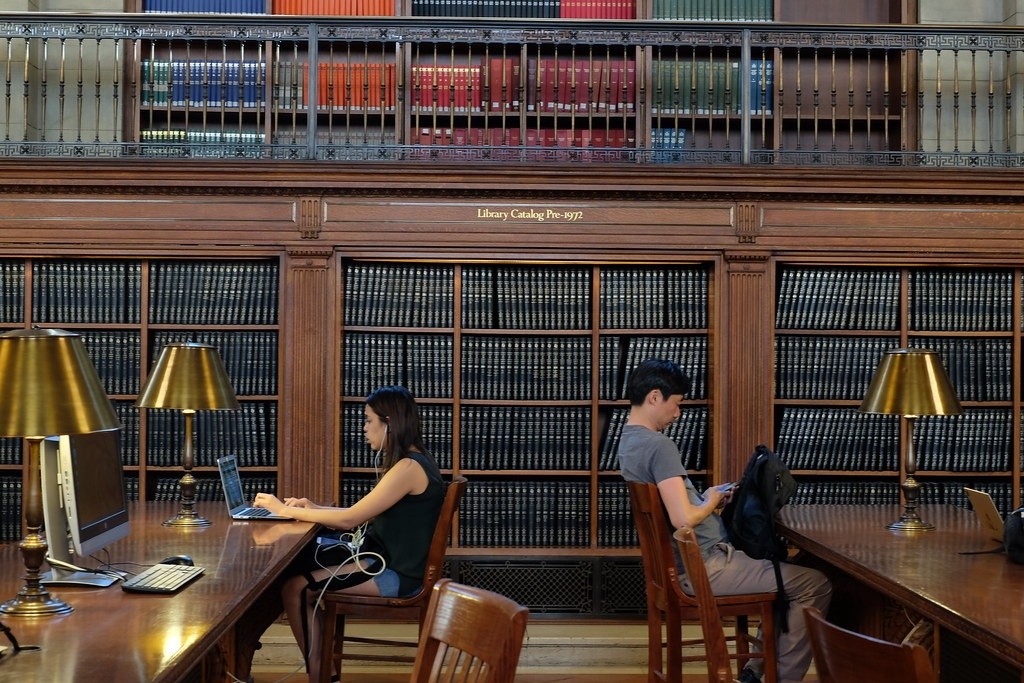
251;385;446;683
618;360;833;683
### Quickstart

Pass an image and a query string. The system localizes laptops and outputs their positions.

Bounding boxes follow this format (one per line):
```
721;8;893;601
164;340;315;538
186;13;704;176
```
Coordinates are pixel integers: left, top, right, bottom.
217;455;292;520
964;487;1006;543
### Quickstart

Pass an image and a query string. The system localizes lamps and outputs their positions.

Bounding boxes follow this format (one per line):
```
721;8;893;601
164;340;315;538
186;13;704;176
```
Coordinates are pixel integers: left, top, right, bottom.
857;349;964;536
0;324;124;625
138;347;240;529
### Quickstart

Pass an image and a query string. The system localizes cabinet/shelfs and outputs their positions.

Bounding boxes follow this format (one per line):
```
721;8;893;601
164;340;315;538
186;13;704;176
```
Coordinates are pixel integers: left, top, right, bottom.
2;251;1024;554
120;0;1024;174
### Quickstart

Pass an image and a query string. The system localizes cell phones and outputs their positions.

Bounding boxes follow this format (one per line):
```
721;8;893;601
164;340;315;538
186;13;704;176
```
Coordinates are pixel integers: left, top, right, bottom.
316;537;356;550
719;481;740;492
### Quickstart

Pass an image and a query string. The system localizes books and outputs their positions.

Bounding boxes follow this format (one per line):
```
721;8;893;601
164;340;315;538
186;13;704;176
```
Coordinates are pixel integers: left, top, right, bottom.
340;255;713;549
137;0;776;176
0;253;283;541
774;264;1024;518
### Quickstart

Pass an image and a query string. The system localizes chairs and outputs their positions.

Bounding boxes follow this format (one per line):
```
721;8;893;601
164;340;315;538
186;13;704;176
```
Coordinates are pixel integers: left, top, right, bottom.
307;477;469;683
626;480;778;683
411;577;529;683
673;523;733;683
803;606;937;683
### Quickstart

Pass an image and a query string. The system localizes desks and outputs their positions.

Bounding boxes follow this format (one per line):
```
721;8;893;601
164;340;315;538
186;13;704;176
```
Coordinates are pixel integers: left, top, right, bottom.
0;501;336;683
778;503;1024;683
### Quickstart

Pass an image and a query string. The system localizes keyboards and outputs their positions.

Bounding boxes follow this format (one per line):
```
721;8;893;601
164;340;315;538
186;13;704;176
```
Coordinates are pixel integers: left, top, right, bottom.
120;563;206;593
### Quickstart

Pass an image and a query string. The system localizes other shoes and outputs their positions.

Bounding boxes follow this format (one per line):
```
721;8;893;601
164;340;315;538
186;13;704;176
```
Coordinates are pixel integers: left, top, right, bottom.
735;667;762;683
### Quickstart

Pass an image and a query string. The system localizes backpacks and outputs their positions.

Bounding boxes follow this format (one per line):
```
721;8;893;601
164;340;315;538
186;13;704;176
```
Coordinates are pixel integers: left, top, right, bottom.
720;445;796;561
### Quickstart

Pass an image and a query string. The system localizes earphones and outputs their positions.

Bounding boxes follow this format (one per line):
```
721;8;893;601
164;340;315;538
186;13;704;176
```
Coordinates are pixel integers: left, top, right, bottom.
385;426;388;433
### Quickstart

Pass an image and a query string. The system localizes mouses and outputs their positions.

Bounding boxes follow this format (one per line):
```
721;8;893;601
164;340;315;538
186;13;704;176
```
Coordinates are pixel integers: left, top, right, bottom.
158;555;194;567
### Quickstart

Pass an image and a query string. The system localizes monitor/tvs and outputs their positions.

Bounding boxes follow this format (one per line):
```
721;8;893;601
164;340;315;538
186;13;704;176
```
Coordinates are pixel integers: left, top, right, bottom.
39;427;131;590
